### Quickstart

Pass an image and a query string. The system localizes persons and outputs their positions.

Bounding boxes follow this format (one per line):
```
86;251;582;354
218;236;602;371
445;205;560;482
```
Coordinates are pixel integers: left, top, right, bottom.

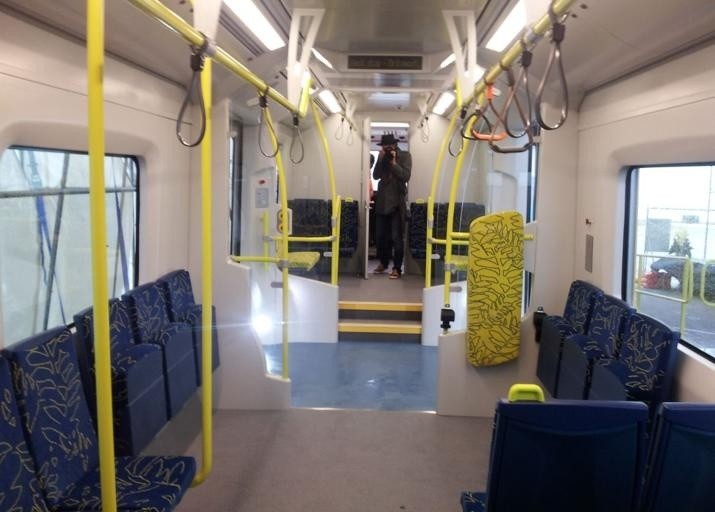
373;135;412;279
369;154;374;197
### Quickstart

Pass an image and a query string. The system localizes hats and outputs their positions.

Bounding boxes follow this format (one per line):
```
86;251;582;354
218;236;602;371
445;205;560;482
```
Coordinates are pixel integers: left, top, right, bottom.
377;134;400;146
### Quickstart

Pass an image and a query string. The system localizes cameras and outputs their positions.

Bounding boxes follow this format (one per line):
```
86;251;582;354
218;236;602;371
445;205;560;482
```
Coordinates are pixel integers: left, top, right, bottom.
384;147;393;159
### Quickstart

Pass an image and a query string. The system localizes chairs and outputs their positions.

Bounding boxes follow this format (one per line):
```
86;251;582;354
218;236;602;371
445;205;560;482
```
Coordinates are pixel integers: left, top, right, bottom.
284;199;359;253
461;280;715;510
0;268;219;512
409;202;484;258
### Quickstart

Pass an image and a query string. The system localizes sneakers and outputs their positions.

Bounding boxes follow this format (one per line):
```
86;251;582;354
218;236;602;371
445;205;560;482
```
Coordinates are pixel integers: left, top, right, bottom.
389;268;401;279
373;263;389;274
